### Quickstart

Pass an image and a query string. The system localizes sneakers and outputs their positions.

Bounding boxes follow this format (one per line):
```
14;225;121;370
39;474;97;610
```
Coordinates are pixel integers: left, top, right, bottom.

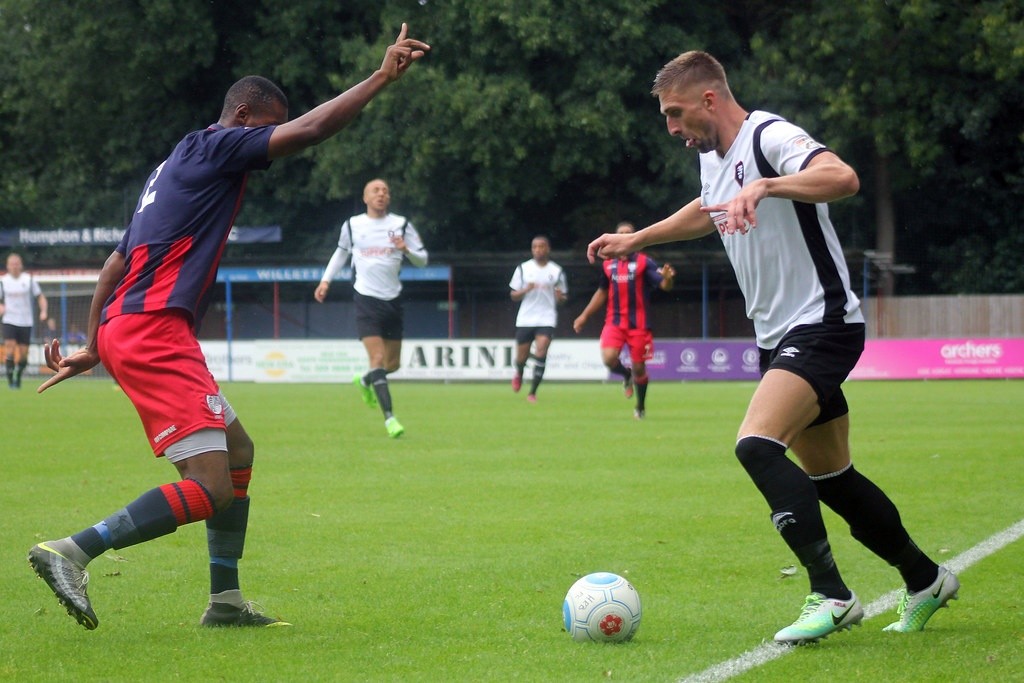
201;601;291;627
773;590;863;646
27;540;98;631
354;374;378;408
385;418;404;439
882;566;960;633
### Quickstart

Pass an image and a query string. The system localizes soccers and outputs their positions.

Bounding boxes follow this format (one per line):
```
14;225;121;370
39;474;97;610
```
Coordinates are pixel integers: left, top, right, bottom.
560;572;642;642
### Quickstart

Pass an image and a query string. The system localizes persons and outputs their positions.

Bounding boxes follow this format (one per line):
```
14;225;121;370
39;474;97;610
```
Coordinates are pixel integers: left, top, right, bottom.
587;50;960;644
0;253;49;390
574;222;674;419
508;235;568;401
315;179;429;438
27;22;431;631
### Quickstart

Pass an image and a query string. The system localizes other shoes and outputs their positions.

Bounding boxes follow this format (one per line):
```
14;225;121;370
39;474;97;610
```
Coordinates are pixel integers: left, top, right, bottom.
512;371;521;392
528;394;536;403
635;407;644;420
623;367;633;398
